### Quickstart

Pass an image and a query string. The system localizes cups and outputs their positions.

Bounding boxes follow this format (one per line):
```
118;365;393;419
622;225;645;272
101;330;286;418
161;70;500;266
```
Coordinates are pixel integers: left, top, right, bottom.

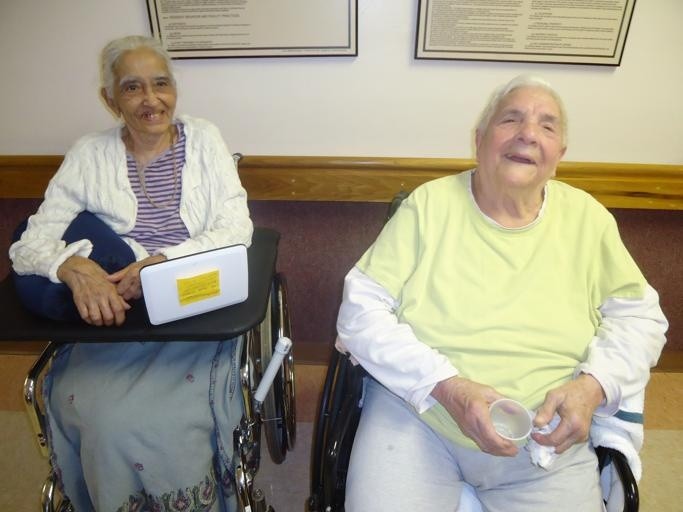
488;398;534;441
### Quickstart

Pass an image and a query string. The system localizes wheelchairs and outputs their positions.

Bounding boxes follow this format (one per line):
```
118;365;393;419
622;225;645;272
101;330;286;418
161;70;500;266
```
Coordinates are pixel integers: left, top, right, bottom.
304;191;641;512
0;153;297;512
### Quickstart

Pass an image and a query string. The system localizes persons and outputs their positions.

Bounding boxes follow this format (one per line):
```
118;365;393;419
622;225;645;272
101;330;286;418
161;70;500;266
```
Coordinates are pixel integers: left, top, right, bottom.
8;33;259;512
333;72;672;511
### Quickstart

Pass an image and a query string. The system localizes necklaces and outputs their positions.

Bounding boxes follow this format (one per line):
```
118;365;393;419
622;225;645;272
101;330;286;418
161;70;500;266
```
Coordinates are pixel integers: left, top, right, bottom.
133;125;176;209
